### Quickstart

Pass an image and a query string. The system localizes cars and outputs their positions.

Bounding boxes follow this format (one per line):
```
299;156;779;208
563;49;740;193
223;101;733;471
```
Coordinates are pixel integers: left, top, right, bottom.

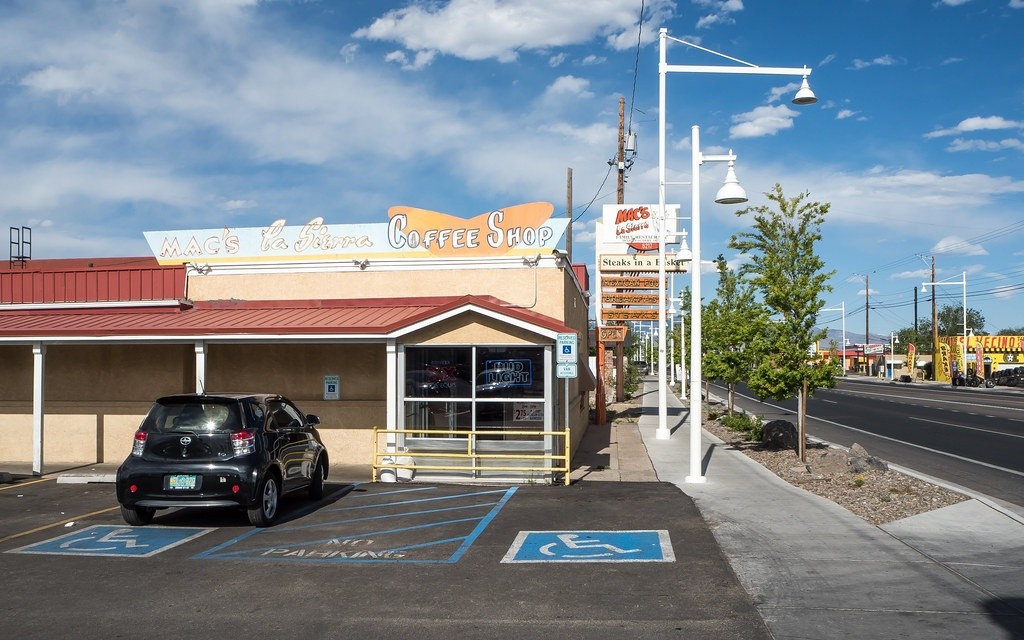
407;359;536;420
116;392;330;529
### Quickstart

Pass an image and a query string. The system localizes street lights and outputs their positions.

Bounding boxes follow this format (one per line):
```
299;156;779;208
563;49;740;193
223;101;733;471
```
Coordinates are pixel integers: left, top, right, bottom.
691;124;750;482
656;26;820;442
813;302;846;376
891;332;899;381
921;271;968;378
